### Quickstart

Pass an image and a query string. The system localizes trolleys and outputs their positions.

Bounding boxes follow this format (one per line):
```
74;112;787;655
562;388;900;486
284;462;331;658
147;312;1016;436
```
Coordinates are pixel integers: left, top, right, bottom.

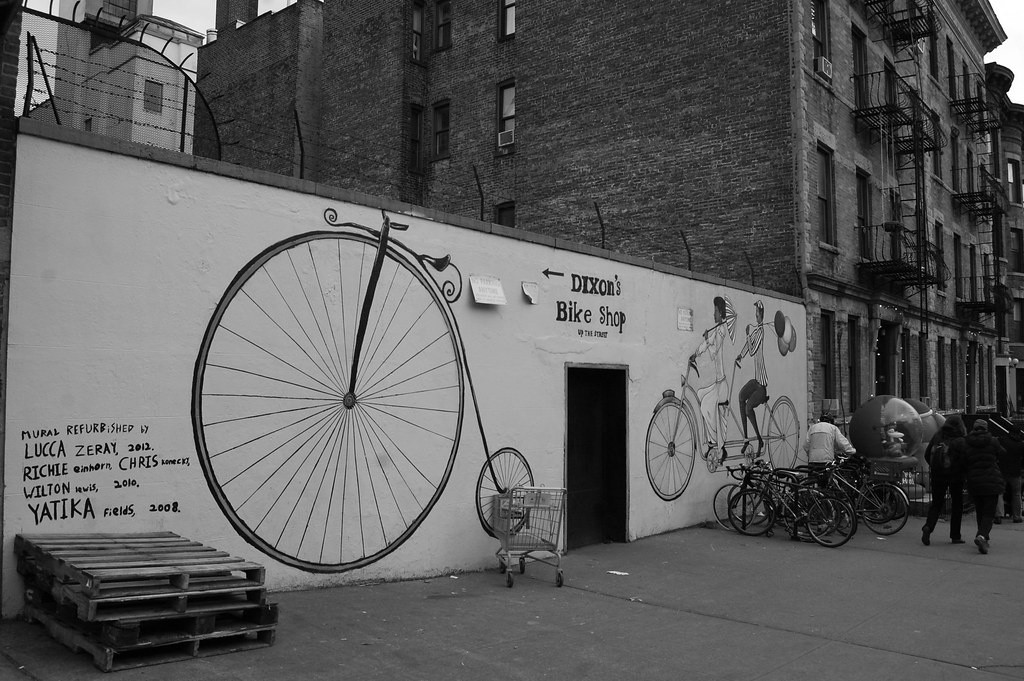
493;483;568;589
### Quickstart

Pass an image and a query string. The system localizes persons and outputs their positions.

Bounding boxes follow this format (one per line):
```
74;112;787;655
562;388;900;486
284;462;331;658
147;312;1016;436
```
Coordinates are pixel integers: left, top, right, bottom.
995;423;1024;526
920;414;970;547
802;410;862;528
962;417;1008;555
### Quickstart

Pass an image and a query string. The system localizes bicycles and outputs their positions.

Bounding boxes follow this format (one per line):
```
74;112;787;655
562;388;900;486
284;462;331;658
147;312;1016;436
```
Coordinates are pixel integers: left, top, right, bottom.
711;440;911;547
191;206;537;574
646;348;801;500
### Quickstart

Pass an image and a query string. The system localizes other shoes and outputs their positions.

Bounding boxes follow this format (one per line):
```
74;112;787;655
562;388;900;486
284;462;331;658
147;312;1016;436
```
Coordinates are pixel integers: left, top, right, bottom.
921;526;930;545
1013;518;1022;523
993;517;1001;524
952;539;965;543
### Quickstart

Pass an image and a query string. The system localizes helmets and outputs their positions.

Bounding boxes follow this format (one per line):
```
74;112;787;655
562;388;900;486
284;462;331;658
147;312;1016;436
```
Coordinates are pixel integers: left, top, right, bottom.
820;412;834;423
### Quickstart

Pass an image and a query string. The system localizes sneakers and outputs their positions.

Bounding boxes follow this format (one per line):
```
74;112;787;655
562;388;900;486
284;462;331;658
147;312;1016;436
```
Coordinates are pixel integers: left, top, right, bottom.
974;537;989;554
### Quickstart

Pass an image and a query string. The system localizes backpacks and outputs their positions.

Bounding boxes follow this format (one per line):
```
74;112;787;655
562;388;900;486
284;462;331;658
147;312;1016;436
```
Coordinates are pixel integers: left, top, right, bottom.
930;434;960;480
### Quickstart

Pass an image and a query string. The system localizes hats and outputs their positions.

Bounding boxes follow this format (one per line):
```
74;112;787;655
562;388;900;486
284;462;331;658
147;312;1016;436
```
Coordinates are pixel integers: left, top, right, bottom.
973;418;988;429
